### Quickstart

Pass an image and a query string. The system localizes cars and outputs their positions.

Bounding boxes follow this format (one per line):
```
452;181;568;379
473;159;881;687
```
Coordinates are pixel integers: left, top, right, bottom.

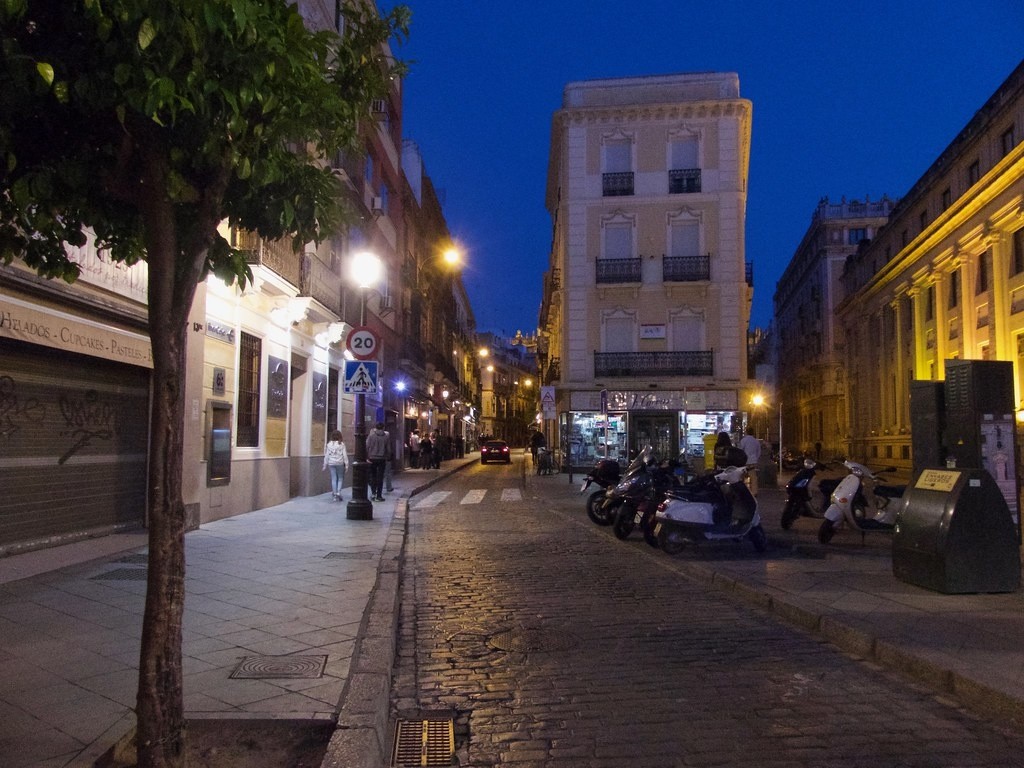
480;439;510;463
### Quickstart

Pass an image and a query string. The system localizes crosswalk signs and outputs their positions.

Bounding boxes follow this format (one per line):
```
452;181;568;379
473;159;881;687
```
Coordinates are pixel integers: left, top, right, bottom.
345;360;379;394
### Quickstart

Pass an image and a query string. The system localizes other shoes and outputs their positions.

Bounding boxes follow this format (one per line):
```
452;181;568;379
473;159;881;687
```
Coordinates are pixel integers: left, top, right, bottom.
375;498;385;501
427;468;429;470
387;488;394;492
423;467;425;469
372;494;377;499
332;493;344;502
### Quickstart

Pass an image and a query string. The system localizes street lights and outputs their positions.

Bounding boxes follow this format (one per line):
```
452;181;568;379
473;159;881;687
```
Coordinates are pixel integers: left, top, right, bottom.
751;393;783;479
346;249;386;521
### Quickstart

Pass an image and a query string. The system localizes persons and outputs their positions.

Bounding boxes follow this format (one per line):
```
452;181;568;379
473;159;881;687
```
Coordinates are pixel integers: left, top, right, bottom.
408;427;445;468
737;428;761;499
531;430;545;466
714;432;748;486
321;430;350;501
366;423;390;501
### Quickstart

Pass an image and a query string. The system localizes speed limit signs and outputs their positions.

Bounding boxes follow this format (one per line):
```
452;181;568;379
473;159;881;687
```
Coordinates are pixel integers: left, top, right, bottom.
346;326;383;362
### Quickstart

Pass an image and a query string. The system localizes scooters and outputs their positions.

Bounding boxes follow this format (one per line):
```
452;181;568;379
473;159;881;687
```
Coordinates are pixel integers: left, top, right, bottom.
816;453;908;546
577;449;724;549
652;465;768;558
780;442;867;532
